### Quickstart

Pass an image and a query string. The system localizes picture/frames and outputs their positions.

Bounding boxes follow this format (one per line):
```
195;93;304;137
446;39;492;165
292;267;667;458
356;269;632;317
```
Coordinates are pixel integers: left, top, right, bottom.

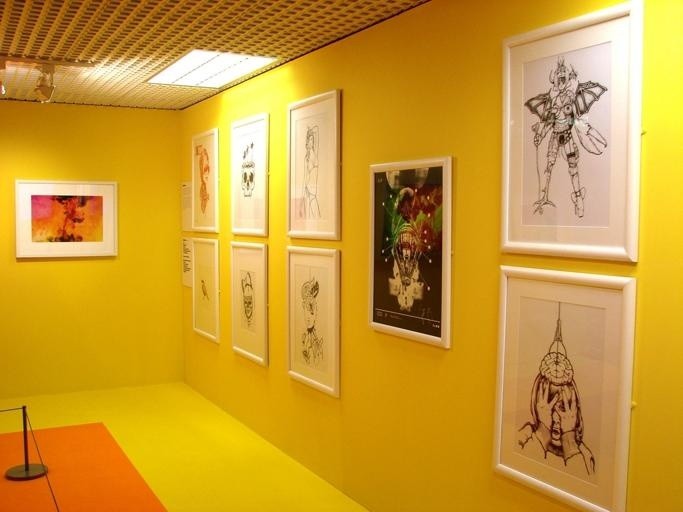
486;264;638;512
284;244;343;400
285;86;340;241
227;240;268;368
366;154;453;352
13;176;119;264
189;127;222;235
495;1;641;268
230;111;269;237
190;238;221;347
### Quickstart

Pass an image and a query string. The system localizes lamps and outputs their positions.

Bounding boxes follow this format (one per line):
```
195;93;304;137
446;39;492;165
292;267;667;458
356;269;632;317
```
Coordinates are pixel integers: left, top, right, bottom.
31;63;54;105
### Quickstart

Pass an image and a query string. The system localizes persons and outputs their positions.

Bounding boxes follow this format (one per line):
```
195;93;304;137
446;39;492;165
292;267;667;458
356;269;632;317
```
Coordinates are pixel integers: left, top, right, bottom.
299;277;324;365
516;302;594;481
239;272;256;330
302;126;323;222
196;145;210;215
